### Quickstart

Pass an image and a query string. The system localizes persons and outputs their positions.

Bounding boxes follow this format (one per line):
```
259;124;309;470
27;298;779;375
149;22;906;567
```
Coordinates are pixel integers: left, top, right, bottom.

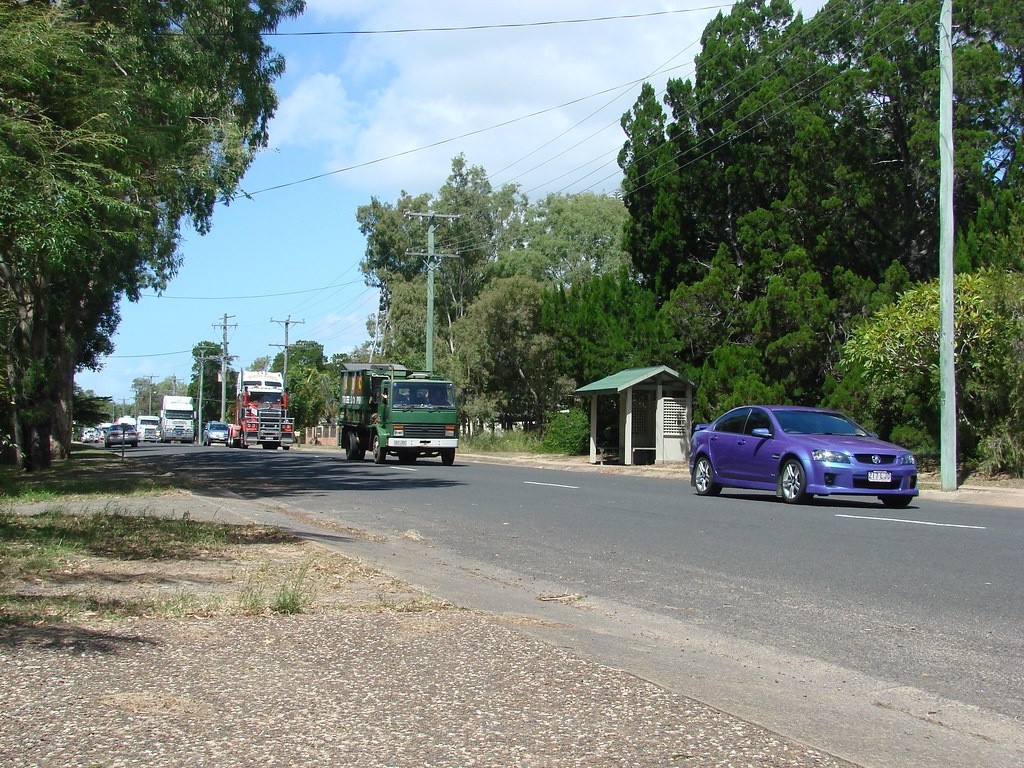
419;389;432;405
382;388;409;405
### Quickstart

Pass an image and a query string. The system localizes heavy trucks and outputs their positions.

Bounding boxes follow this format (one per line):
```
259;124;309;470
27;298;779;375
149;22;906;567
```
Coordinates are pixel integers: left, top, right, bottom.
228;369;295;452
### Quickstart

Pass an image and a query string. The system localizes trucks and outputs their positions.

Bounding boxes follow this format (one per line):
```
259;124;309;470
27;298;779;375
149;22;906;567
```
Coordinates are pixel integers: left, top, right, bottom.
159;394;195;445
333;367;458;468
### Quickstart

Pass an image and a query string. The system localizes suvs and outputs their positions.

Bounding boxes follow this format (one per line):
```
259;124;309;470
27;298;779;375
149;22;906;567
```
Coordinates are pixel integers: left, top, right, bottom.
201;421;228;447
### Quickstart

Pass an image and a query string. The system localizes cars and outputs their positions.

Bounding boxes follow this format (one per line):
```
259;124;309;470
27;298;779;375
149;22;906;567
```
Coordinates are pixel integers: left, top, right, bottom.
687;404;920;513
82;415;160;447
